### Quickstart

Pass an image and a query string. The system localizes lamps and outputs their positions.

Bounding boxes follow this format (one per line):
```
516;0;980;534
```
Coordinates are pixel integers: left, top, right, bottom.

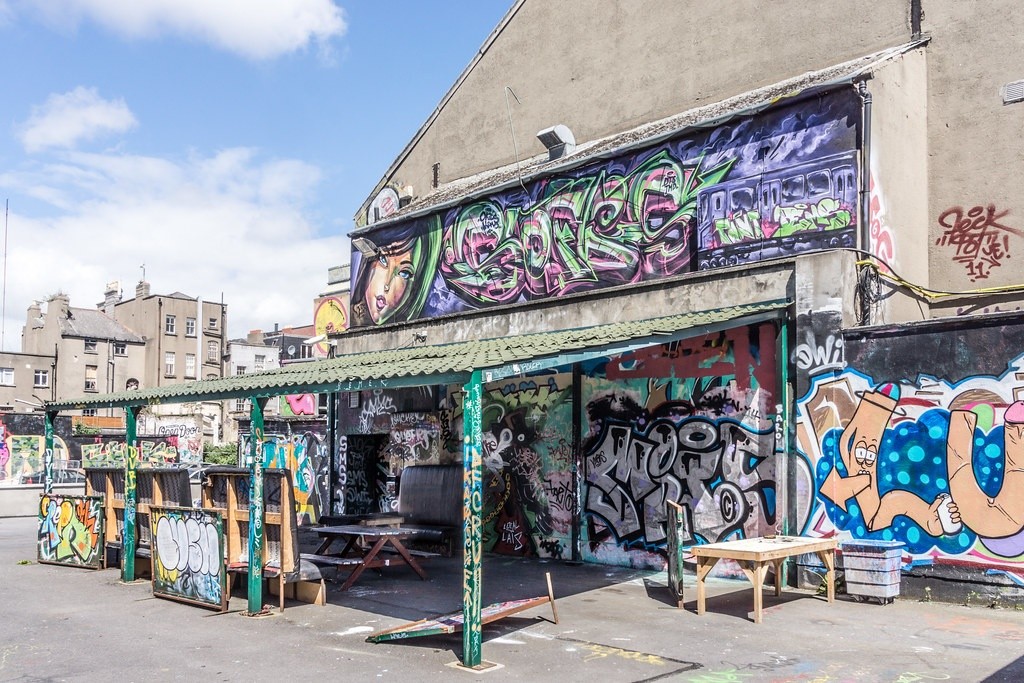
352;237;381;262
536;124;577;162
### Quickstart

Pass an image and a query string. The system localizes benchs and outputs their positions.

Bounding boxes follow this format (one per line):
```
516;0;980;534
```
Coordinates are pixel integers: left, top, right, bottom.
200;466;338;613
83;464;193;570
359;546;442;557
299;553;365;565
387;464;463;558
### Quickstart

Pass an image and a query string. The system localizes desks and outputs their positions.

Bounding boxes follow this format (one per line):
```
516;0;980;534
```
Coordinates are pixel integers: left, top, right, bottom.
691;535;837;623
309;524;426;559
318;514;404;554
